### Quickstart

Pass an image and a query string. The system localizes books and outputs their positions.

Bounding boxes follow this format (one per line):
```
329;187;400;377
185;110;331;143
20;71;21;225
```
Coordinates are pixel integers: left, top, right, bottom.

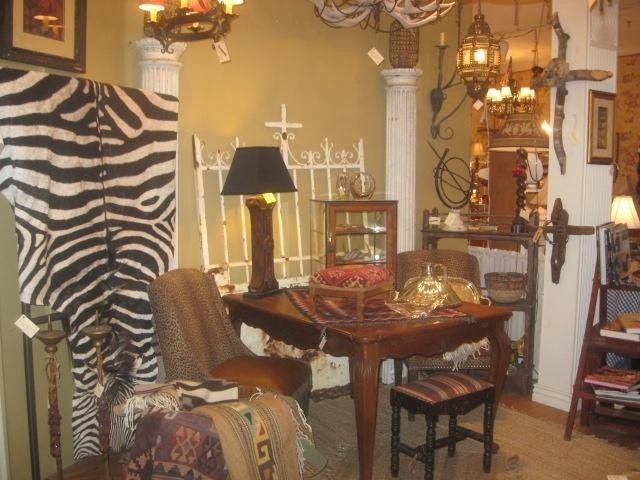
591;379;640;404
617;312;640;333
583;365;640;389
599;316;640;344
604;223;633;288
594;220;616;287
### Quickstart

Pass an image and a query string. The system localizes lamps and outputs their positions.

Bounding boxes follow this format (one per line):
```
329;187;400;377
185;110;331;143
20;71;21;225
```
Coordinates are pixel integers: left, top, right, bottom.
611;191;640;230
218;145;295;296
480;80;540;113
457;1;500;104
136;0;243;50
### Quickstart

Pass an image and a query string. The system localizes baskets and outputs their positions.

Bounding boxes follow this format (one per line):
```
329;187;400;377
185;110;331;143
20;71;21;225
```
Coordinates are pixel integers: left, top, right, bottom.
483;271;528;303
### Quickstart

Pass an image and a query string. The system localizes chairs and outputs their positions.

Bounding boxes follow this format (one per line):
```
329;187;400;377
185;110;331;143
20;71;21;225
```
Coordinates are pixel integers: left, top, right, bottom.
146;263;317;419
390;247;495;421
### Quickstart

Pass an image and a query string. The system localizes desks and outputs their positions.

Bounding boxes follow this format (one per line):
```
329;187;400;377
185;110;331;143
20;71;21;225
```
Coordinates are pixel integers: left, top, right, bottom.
218;280;516;480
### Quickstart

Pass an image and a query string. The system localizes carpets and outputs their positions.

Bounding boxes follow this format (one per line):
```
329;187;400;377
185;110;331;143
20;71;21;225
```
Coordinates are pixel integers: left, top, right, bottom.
303;395;639;480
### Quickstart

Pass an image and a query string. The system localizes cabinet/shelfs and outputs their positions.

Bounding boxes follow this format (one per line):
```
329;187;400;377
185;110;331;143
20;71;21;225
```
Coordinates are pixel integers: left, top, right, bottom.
307;196;399;286
560;269;640;440
420;206;538;401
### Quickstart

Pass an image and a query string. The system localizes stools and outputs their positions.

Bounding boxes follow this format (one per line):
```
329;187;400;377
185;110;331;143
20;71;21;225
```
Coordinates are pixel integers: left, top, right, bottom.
385;368;497;479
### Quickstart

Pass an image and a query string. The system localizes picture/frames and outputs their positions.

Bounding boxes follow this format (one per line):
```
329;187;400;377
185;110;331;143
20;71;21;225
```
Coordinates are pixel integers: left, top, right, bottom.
585;90;617;168
0;1;91;72
